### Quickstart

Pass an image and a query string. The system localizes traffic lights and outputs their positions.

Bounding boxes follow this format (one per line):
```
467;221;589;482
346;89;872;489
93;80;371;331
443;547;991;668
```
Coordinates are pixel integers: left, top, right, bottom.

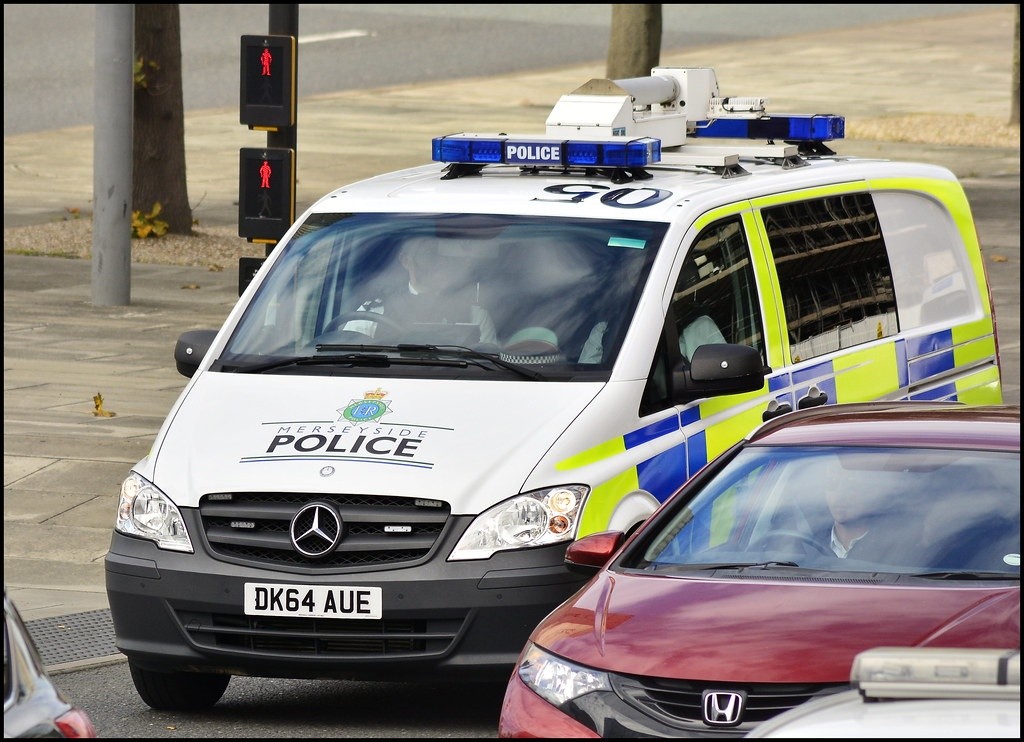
239;148;295;240
239;35;294;127
239;257;268;297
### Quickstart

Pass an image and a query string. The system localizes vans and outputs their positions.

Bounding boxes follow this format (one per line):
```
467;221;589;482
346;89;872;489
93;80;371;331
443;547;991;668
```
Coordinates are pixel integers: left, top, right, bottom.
105;65;1004;714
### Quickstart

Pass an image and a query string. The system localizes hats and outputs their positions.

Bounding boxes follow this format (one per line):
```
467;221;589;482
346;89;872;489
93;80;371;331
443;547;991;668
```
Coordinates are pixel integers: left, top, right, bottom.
499;326;565;364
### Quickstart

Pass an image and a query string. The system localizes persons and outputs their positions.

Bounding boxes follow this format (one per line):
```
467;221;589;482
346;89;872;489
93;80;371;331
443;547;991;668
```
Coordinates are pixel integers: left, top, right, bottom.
774;461;895;564
573;232;728;371
342;232;497;349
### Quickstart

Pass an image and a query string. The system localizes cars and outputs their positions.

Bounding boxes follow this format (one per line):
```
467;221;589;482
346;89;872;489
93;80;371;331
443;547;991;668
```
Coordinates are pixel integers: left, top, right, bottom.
498;401;1019;739
745;646;1019;737
4;593;96;738
